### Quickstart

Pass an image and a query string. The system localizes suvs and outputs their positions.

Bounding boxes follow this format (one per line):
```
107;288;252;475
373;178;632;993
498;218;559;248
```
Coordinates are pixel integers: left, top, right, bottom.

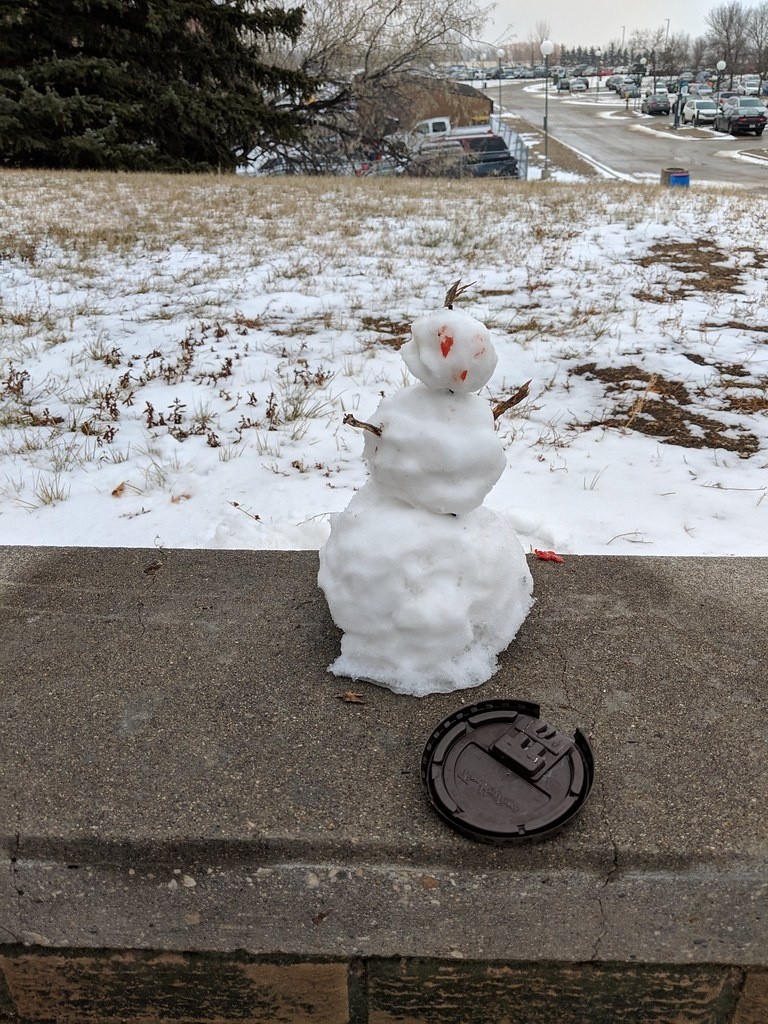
616;79;637;95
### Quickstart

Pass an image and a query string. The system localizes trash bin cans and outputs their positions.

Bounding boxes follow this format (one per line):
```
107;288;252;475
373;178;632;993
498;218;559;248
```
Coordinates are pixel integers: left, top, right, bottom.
661;167;689;189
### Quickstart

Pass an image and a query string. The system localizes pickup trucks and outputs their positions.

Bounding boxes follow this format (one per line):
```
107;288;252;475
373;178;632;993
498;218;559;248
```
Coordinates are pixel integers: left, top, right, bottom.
626;73;642;87
254;112;520;181
627;63;647;77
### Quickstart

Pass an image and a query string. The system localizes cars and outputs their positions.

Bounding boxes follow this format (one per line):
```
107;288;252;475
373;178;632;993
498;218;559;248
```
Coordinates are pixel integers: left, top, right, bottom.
570;79;587;92
642;94;671;115
605;76;624;87
664;71;768;97
647;82;668;98
709;92;740;109
620;84;642;99
681;99;723;128
575;78;590;88
722;94;768;125
714;107;767;136
556;79;569;90
672;94;702;115
431;61;627;78
609;78;625;91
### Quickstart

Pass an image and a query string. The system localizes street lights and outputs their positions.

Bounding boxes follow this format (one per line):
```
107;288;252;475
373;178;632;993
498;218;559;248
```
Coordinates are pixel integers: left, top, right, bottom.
496;48;505;121
470;58;476;88
479;52;489;95
539;40;554;174
637;57;648;109
714;60;726;132
593;50;602;103
